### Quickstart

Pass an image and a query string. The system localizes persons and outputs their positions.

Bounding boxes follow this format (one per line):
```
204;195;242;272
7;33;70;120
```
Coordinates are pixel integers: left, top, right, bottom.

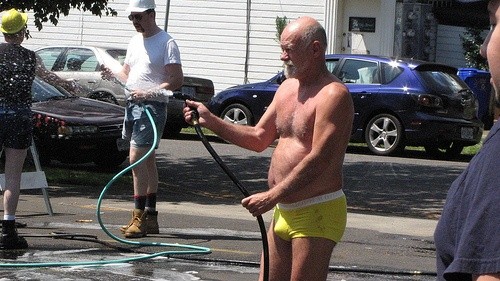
434;0;500;281
0;8;72;249
67;58;82;71
183;16;354;281
101;0;184;238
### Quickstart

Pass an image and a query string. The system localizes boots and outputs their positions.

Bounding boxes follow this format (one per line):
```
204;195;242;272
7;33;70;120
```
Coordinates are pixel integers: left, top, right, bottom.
0;220;29;249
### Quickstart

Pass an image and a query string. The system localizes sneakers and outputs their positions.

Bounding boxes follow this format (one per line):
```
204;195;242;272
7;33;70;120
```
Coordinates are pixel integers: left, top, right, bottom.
120;210;159;234
125;209;146;237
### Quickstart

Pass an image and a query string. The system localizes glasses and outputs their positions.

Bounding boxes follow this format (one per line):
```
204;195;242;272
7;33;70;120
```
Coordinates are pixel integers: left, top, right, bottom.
128;12;148;21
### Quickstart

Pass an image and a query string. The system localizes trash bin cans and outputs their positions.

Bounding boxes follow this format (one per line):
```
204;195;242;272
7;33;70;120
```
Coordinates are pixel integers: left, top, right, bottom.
458;68;491;117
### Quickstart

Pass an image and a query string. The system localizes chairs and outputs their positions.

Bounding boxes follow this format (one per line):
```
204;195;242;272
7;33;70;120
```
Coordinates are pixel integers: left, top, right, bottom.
344;65;360;84
66;57;82;71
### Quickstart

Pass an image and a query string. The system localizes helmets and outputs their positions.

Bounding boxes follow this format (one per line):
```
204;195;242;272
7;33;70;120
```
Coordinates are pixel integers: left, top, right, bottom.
0;9;28;33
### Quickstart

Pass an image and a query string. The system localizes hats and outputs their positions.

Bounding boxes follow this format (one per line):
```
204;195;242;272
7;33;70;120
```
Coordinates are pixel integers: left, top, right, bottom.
127;0;155;12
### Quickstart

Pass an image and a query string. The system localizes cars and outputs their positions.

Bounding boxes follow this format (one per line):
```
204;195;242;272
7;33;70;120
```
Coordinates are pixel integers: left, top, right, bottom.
27;45;215;168
205;53;484;160
